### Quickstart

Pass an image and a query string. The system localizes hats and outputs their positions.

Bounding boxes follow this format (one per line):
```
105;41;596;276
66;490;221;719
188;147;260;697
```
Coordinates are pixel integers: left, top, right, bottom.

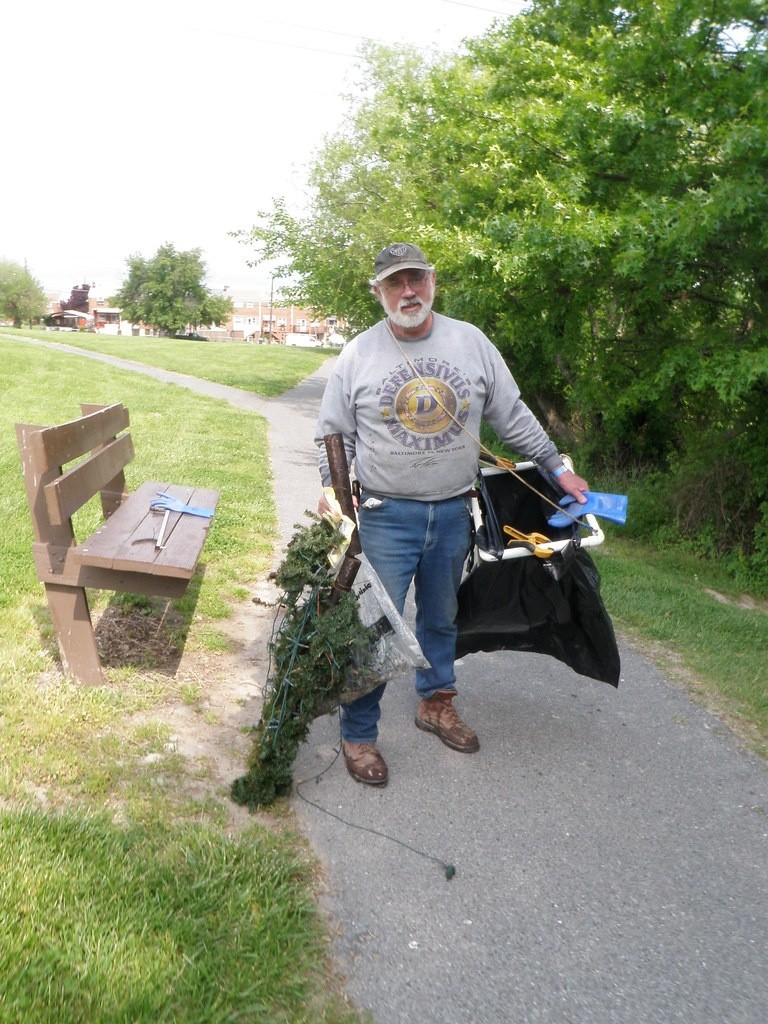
374;243;429;282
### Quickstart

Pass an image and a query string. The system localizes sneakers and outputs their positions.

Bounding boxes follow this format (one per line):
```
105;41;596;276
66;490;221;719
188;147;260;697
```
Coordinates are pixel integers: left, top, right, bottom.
415;688;480;754
342;736;390;784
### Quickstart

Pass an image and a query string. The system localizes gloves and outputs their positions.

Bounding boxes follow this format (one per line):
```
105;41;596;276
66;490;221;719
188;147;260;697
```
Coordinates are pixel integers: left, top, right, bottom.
149;491;215;519
549;492;629;527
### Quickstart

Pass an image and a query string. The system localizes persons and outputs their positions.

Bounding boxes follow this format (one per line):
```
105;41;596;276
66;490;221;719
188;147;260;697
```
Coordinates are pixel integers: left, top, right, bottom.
315;242;591;784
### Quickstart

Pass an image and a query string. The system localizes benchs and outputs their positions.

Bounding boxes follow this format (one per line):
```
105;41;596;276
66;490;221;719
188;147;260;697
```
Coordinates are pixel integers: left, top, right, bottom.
13;402;221;686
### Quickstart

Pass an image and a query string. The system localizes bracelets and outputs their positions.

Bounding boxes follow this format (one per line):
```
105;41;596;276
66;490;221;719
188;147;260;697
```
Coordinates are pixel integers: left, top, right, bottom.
552;464;568;479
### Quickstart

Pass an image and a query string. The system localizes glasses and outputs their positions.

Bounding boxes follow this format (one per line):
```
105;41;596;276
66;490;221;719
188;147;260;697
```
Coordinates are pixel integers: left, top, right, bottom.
377;271;428;294
